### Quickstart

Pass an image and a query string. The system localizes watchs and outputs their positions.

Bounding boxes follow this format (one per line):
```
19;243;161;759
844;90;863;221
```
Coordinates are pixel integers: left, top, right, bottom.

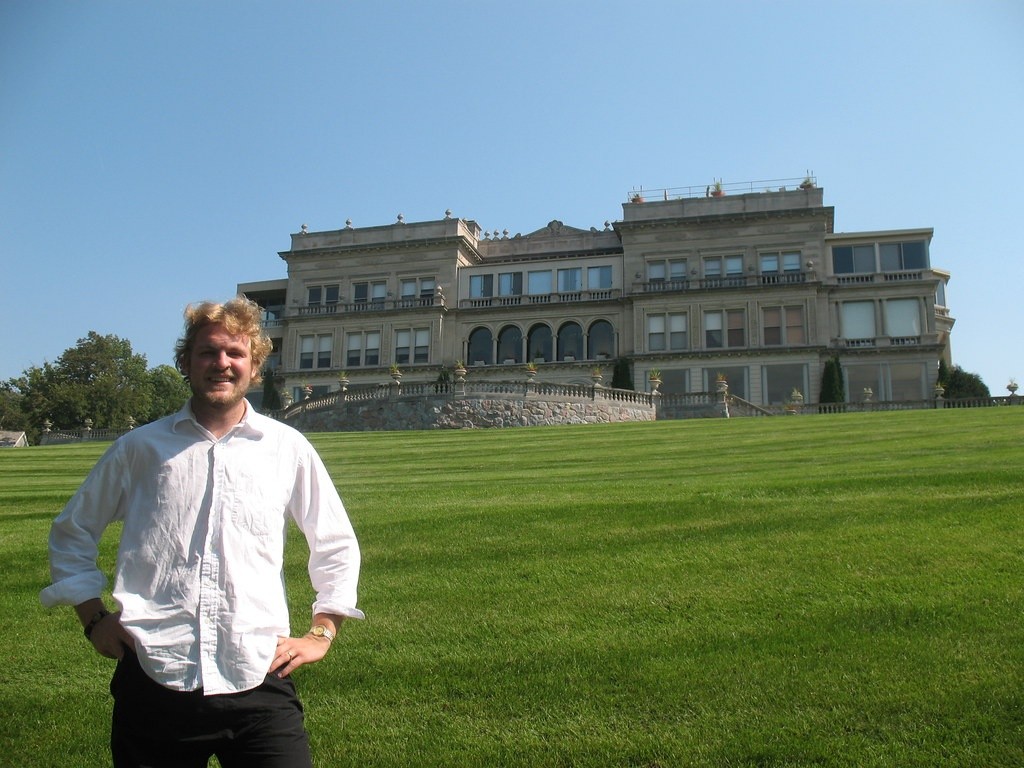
310;625;335;642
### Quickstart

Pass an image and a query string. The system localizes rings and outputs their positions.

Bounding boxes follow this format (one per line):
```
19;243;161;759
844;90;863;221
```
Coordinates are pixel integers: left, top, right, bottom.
286;651;292;662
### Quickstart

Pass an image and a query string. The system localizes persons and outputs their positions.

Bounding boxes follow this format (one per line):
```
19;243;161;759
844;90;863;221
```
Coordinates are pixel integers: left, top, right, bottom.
40;296;364;768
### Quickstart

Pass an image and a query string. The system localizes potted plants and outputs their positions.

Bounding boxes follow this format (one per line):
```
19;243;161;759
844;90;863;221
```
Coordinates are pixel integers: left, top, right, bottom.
711;182;725;197
525;361;538;381
800;179;817;189
85;419;93;430
337;371;349;390
864;387;873;401
388;362;402;383
278;387;293;411
791;387;804;403
935;382;946;400
1006;377;1018;396
454;360;467;381
592;367;603;385
716;373;729;391
125;415;135;429
44;419;52;431
648;368;662;392
631;193;646;203
302;378;313;400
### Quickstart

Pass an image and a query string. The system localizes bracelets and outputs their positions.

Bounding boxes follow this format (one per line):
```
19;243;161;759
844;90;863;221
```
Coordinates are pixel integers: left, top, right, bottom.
84;610;110;640
